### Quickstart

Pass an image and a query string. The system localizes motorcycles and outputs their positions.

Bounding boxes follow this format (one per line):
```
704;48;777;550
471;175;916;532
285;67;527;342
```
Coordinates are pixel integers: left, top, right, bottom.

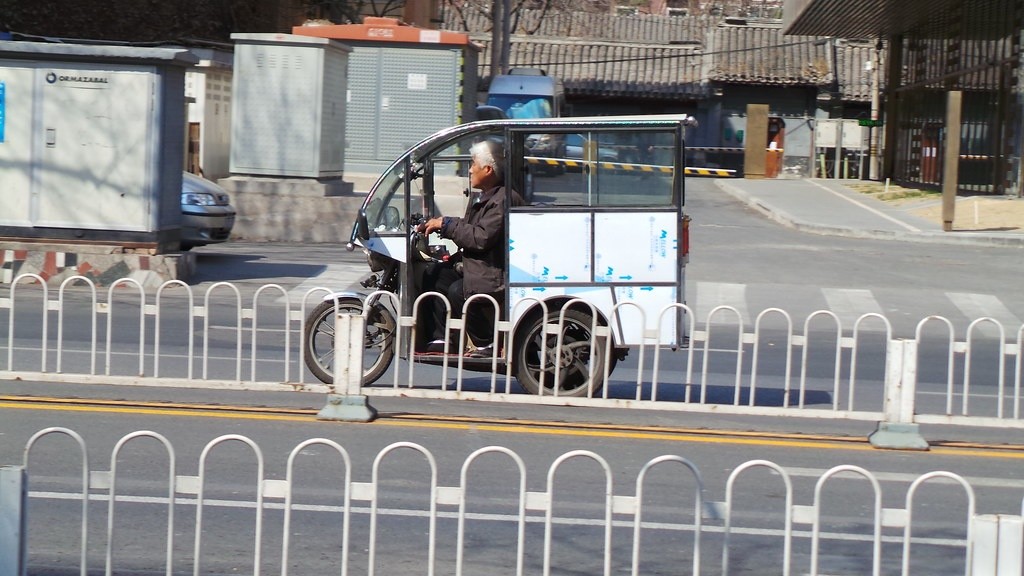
303;112;698;398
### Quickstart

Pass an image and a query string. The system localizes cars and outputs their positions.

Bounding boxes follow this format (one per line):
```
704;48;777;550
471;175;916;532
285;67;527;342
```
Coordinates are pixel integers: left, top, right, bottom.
182;170;236;249
564;129;618;164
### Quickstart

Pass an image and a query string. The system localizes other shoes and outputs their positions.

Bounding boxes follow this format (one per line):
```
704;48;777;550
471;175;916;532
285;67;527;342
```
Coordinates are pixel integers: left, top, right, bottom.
414;341;454;355
471;348;496;358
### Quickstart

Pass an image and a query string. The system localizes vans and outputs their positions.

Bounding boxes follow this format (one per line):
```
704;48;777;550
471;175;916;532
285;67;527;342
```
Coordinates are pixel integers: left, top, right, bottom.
943;101;1010;183
485;68;566;174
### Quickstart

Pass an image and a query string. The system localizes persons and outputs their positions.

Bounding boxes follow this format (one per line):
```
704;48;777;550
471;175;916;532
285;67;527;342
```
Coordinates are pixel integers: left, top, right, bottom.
415;140;526;357
